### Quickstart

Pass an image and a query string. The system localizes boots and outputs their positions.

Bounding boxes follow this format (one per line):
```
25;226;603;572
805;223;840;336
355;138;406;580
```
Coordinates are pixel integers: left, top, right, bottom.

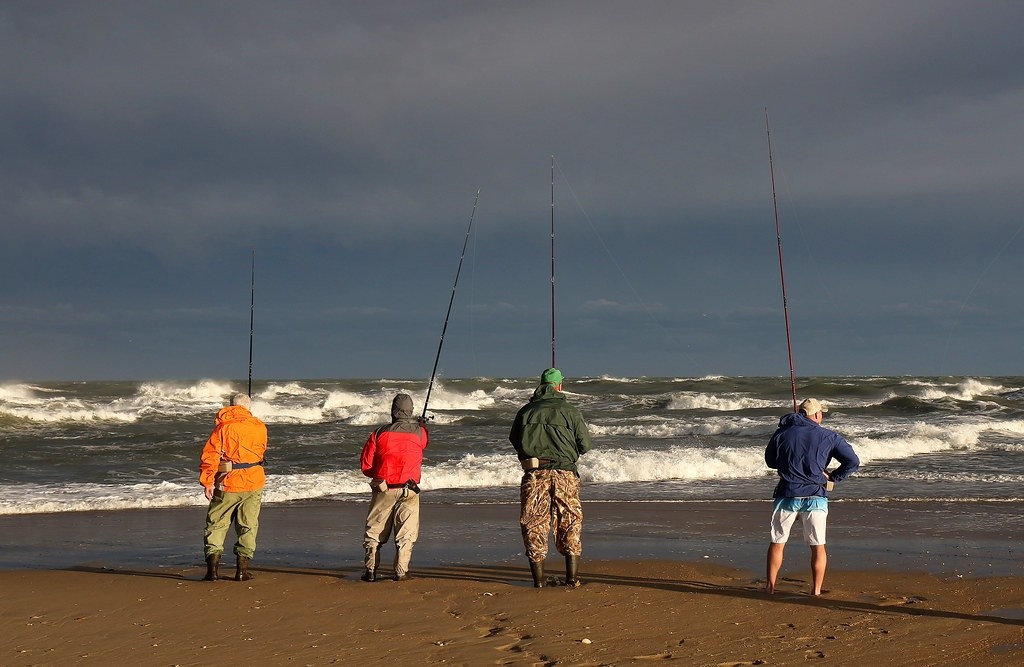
201;553;221;581
530;560;547;588
235;555;255;581
565;554;582;587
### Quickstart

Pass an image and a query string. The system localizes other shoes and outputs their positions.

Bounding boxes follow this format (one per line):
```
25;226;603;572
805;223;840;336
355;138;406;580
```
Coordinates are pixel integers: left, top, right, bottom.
394;572;412;581
361;571;376;581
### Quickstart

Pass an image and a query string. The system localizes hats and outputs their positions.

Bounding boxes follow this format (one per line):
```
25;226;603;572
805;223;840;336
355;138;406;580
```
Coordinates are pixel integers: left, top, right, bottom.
540;368;565;387
800;396;828;416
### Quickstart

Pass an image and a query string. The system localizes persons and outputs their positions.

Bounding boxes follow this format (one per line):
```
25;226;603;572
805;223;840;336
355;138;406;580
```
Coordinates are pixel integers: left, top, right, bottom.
360;394;427;583
509;366;590;587
765;398;859;595
198;394;267;582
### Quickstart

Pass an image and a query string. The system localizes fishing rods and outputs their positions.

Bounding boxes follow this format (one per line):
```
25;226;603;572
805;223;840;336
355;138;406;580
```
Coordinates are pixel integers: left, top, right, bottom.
549;155;559;370
414;185;483;427
764;106;799;414
248;249;256;400
